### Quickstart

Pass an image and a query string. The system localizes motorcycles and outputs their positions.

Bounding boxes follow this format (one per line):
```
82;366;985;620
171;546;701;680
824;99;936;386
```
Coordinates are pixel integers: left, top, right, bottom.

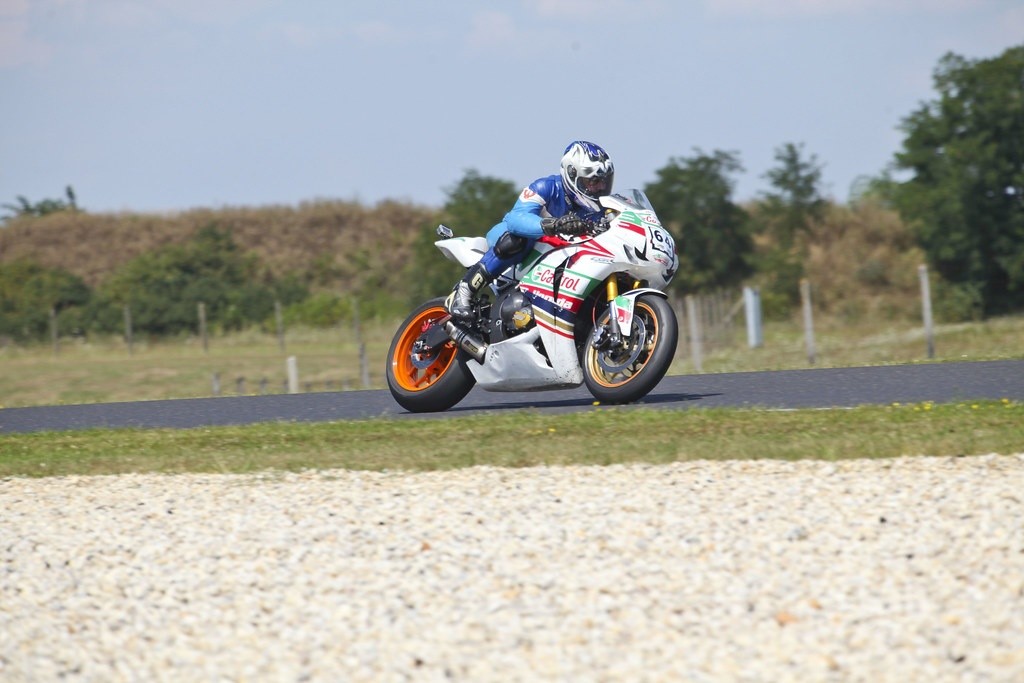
385;189;679;414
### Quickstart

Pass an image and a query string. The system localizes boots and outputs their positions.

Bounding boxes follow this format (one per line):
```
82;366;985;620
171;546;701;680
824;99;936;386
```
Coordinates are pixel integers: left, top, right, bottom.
443;262;493;322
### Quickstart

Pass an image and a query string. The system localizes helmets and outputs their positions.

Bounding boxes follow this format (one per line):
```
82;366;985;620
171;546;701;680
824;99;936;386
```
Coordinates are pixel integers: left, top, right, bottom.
560;141;615;213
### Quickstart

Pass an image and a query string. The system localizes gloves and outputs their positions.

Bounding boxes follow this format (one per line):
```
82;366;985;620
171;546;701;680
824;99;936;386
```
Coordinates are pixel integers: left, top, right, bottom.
541;215;584;236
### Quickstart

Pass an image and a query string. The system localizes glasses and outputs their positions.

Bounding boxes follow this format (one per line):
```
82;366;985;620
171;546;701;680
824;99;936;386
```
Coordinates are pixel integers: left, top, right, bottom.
583;176;610;185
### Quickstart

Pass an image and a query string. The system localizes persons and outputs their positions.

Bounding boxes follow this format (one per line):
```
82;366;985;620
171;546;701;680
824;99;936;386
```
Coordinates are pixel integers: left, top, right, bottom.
443;141;630;321
